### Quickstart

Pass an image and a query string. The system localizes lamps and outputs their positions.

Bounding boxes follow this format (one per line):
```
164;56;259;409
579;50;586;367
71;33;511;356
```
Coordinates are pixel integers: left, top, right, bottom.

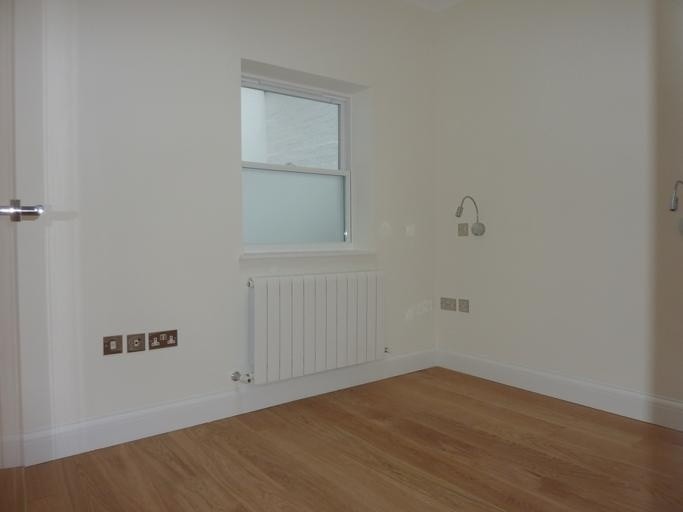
455;195;485;237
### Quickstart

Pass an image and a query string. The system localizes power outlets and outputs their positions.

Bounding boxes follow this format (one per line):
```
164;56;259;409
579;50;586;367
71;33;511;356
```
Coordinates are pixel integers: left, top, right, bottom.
102;336;122;355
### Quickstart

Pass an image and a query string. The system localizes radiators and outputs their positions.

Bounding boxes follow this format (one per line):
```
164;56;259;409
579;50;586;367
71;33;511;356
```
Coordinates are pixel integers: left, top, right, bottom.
242;271;386;386
